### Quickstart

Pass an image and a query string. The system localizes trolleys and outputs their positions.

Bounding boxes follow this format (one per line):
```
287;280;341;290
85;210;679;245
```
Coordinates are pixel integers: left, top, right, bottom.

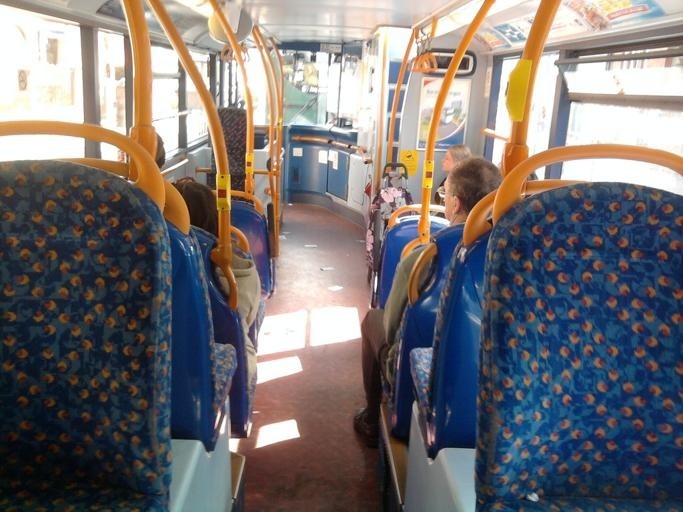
365;162;413;286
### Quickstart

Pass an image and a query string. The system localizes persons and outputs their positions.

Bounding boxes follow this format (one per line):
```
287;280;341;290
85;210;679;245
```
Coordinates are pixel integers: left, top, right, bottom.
352;154;500;449
164;175;262;419
433;141;475;208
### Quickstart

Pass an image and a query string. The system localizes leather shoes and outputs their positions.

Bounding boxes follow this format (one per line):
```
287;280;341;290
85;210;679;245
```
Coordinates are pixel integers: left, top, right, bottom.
355;407;380;446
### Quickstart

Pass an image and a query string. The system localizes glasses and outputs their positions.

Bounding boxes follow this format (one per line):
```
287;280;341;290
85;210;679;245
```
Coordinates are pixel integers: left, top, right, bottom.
437;186;454;198
